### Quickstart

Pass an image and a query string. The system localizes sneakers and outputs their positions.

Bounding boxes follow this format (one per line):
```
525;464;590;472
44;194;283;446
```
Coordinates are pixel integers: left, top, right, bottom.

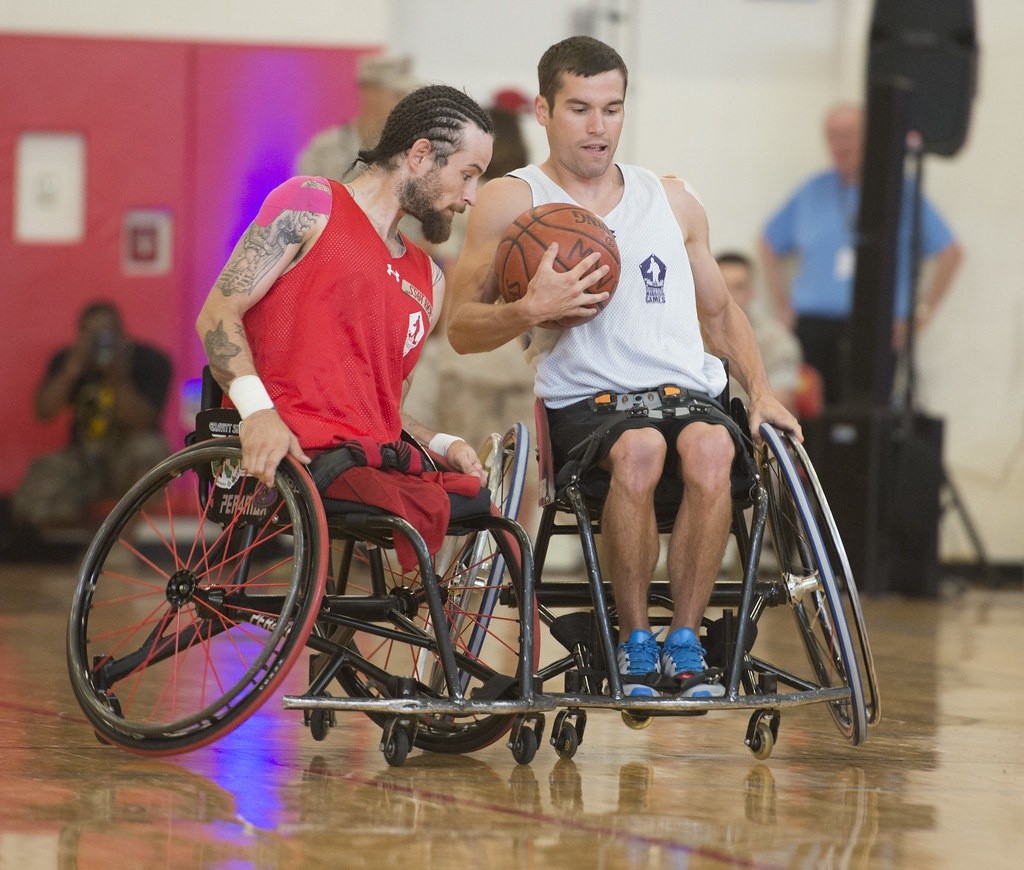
659;627;726;698
601;626;665;697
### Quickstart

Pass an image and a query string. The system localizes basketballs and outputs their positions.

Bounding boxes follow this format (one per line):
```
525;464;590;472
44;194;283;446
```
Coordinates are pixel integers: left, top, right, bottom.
493;201;623;332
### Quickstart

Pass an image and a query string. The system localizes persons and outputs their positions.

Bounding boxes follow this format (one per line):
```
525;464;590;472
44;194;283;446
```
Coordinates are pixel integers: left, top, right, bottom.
196;83;495;574
446;37;805;699
391;104;543;536
714;248;803;410
9;298;174;548
761;103;963;407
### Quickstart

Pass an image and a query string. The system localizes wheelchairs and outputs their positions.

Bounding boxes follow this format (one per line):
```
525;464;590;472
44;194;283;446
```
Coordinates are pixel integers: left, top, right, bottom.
67;365;556;767
417;359;881;759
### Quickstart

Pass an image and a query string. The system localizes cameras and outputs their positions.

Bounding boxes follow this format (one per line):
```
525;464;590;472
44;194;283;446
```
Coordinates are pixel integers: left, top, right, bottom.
92;333;115;359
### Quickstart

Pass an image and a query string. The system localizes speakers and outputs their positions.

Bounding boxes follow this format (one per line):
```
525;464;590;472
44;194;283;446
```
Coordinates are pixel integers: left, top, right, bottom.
865;0;976;157
801;405;946;603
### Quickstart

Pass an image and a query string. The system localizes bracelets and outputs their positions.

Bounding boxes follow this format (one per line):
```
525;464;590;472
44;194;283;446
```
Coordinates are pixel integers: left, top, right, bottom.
228;376;275;421
429;433;467;456
